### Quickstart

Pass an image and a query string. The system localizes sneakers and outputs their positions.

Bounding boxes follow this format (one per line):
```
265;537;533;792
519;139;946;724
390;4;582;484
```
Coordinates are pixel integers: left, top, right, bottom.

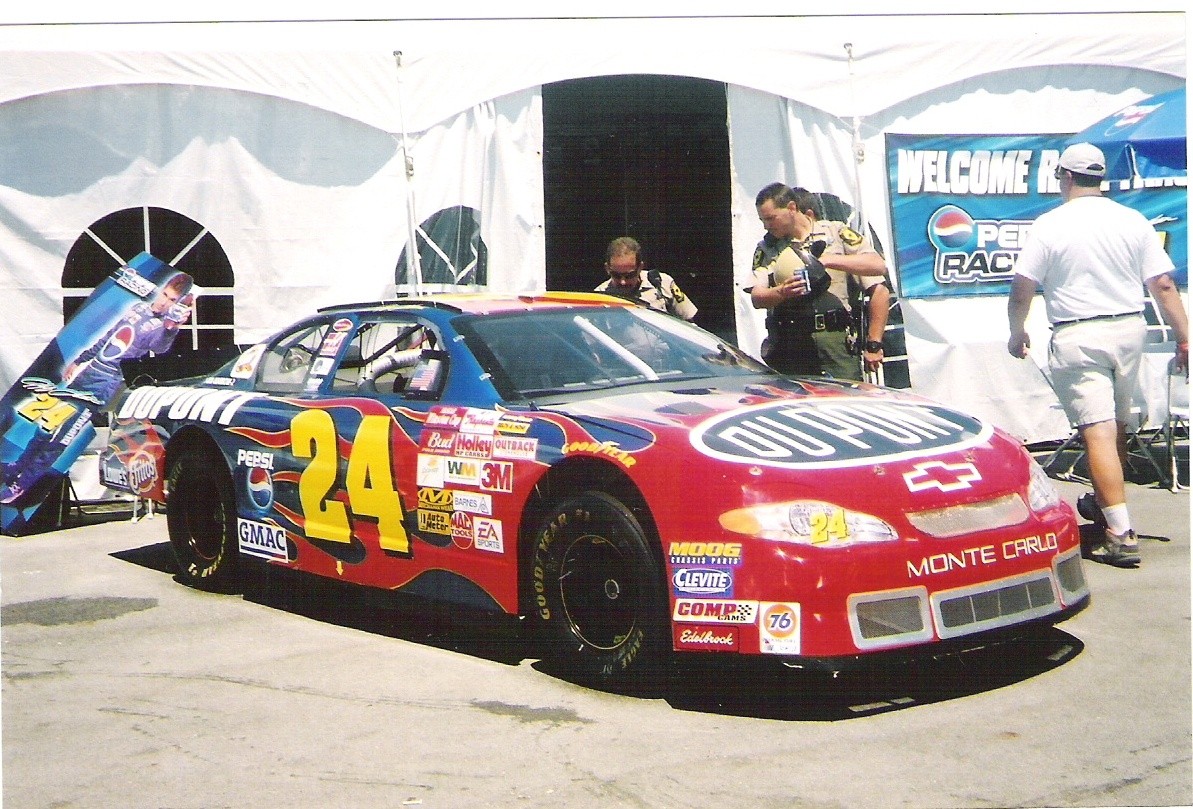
0;462;25;485
0;482;25;503
1081;529;1141;566
1077;493;1109;527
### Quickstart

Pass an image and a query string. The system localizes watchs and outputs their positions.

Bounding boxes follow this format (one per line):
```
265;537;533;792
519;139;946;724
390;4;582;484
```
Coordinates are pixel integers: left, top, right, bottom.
865;341;883;354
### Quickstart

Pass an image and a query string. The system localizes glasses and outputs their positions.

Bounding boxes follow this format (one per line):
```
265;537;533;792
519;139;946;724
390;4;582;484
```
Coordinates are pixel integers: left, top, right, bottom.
608;260;641;279
1054;168;1065;179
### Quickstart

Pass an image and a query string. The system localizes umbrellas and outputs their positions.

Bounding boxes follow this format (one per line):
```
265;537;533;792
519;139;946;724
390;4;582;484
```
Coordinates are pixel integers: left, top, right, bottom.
1059;89;1186;141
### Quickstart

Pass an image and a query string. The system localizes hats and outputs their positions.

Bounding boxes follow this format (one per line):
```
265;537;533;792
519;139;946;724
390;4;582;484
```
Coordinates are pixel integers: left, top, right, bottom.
1054;142;1106;177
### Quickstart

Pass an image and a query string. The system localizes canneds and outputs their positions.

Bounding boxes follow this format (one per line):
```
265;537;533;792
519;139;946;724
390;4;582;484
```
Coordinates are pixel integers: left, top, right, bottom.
792;269;811;295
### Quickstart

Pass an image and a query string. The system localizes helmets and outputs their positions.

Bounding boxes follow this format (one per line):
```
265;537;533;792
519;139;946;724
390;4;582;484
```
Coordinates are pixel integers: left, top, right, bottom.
773;246;833;301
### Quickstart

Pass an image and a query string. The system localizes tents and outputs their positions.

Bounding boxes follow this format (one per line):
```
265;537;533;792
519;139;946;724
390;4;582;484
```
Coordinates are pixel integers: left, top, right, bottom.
0;0;1191;505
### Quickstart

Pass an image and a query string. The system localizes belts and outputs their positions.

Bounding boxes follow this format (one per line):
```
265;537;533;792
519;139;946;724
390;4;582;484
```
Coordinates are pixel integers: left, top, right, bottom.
1056;311;1140;328
765;309;849;330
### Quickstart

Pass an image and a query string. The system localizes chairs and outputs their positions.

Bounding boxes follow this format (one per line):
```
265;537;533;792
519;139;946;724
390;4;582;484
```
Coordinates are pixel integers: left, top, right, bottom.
1024;340;1191;496
0;406;97;538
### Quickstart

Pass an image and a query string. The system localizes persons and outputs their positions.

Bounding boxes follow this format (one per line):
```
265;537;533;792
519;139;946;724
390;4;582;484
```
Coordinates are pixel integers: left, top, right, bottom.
591;237;697;381
789;184;893;375
743;181;888;383
1006;141;1186;565
1;273;194;507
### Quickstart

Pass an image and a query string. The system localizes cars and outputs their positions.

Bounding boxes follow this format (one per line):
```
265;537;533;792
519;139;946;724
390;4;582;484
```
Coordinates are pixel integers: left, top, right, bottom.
92;285;1090;686
